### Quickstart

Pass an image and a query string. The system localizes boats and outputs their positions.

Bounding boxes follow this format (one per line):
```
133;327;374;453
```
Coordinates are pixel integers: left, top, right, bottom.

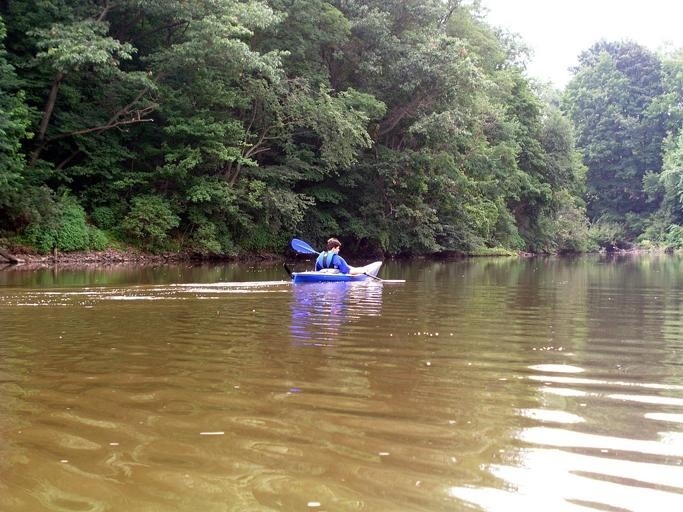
281;259;382;283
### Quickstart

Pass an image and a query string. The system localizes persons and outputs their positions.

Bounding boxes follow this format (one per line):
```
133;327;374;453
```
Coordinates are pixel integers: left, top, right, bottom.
315;238;366;275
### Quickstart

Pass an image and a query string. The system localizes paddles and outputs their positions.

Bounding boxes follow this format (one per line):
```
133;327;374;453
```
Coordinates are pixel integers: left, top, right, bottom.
292;239;383;281
